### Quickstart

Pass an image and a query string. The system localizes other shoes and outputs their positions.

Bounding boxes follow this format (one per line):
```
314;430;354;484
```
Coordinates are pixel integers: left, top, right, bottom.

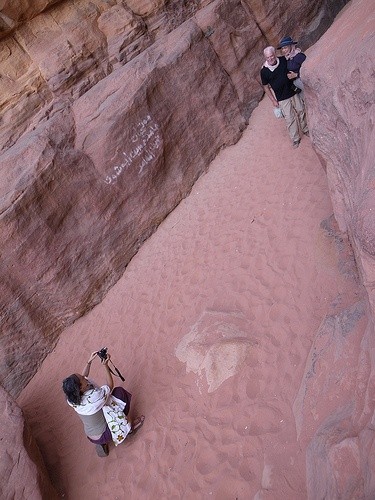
95;443;109;457
304;129;310;137
128;414;145;436
292;138;301;148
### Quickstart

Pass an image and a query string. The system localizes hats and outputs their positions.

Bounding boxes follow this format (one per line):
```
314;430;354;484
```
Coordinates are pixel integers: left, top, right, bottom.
277;36;298;50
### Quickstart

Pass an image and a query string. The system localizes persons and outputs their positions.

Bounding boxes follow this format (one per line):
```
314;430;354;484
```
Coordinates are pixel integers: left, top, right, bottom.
260;47;310;148
62;350;144;457
276;36;307;90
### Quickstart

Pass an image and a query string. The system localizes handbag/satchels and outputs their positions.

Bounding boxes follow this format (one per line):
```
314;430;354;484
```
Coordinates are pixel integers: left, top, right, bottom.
273;106;282;118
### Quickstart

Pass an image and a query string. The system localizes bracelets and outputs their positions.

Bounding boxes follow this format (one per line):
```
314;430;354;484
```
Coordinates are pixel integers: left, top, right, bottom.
87;361;91;365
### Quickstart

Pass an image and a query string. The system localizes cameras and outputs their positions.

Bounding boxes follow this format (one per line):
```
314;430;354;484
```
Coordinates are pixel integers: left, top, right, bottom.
96;347;108;361
